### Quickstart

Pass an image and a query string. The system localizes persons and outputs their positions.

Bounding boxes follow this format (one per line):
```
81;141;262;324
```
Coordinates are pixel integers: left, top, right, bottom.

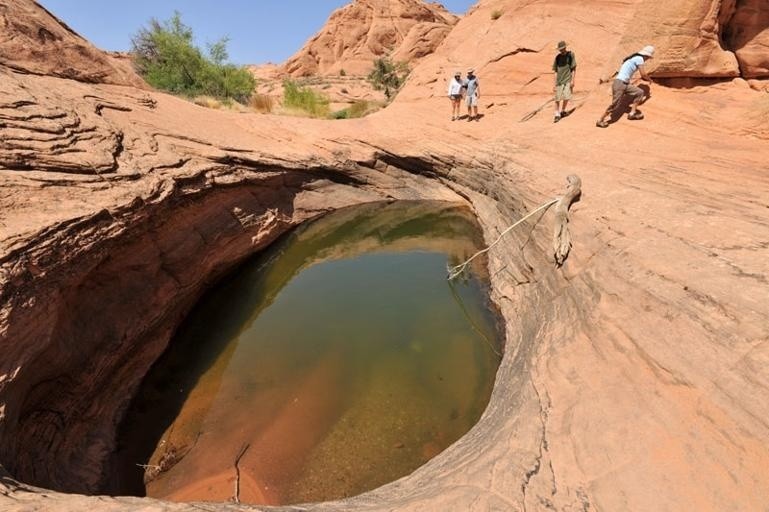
594;44;655;127
447;72;465;119
553;40;577;122
463;68;482;122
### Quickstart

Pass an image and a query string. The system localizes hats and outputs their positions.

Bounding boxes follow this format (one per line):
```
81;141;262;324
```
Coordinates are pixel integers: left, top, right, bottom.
467;67;473;73
555;41;570;51
638;45;655;60
455;72;461;77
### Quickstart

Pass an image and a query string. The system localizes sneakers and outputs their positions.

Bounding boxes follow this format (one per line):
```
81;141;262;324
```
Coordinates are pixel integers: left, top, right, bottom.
452;115;478;122
554;111;568;122
628;112;644;120
596;119;609;128
